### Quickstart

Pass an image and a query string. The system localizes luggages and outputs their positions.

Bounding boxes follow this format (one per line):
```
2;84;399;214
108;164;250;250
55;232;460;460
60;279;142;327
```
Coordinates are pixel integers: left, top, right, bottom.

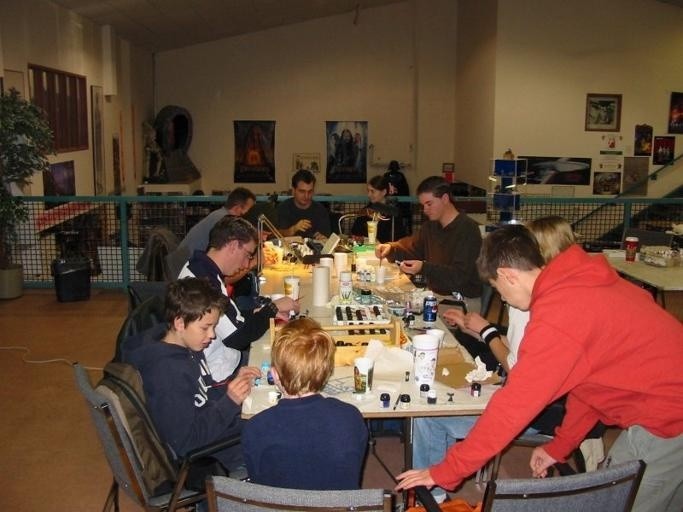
51;231;91;301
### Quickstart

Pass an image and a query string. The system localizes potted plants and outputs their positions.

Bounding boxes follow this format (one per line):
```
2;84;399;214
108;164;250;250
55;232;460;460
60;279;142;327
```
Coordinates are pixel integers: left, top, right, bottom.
0;87;57;299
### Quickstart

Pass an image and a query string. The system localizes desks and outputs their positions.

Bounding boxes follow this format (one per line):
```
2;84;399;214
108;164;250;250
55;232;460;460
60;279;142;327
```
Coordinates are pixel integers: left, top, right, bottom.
236;233;503;512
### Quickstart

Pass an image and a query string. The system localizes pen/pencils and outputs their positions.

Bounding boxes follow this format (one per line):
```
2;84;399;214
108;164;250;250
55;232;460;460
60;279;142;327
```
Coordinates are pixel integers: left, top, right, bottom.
263;240;264;248
204;380;231;389
408;328;426;331
395;260;400;264
380;246;383;267
393;392;402;410
295;295;305;302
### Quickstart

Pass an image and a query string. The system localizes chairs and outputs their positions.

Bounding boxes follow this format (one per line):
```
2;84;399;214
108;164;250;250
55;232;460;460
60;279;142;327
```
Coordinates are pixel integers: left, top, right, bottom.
74;205;683;310
491;424;590;478
393;454;646;512
205;471;399;511
76;363;210;512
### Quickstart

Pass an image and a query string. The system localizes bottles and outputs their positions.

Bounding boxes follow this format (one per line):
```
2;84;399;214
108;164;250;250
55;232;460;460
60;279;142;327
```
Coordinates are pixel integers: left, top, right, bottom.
339;264;372;305
503;147;514;160
380;383;481;409
402;315;415;329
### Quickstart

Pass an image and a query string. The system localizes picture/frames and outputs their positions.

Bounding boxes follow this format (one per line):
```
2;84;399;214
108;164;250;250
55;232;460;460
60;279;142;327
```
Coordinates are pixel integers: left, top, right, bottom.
585;94;623;132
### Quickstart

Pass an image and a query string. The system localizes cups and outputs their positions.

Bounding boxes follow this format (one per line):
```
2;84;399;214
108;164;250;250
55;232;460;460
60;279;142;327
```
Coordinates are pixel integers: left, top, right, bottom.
367;221;378;244
283;275;300;301
312;252;347;306
412;329;445;389
353;357;375;394
375;265;385;284
625;236;640;261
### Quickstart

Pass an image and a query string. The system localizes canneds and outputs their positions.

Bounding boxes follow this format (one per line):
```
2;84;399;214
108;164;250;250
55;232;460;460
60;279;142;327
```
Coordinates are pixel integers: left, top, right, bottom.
423;296;437;321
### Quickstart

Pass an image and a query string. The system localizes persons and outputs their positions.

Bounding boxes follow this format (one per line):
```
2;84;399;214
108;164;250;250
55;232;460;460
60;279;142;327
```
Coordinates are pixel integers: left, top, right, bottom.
335;129;358;168
179;189;271;257
327;134;339;171
375;175;482;331
393;225;683;512
239;316;370;490
383;160;410;218
223;202;278;310
263;170;332;240
175;214;304;383
119;276;263;512
354;133;368;168
349;177;406;243
411;215;576;508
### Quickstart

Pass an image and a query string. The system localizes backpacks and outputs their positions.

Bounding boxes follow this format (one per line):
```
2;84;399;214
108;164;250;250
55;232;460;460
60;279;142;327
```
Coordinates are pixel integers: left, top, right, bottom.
98;360;189;498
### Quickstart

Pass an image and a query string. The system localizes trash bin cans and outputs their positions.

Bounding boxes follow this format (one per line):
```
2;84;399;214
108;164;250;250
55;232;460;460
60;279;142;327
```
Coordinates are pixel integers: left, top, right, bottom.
52;257;91;302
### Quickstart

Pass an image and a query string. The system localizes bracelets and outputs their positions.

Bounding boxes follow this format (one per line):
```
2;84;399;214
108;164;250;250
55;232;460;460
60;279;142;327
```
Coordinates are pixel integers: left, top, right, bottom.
480;323;502;346
